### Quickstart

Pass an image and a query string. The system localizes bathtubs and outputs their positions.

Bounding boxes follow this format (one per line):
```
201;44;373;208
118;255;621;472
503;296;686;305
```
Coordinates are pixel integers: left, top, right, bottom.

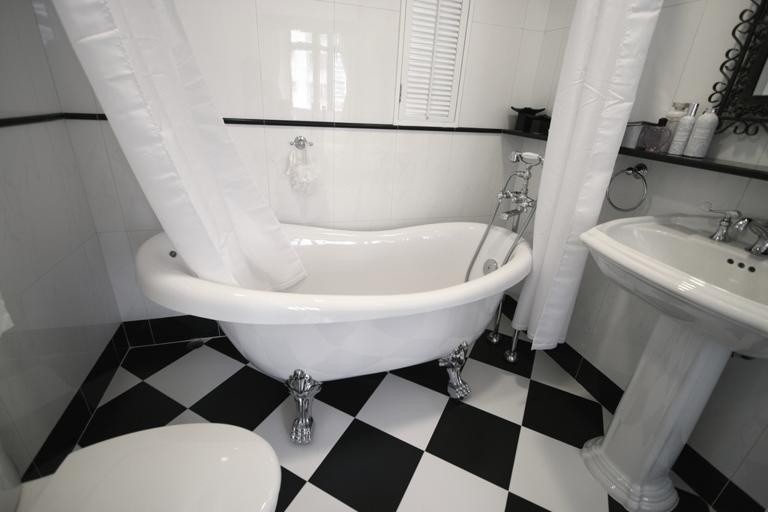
133;222;533;444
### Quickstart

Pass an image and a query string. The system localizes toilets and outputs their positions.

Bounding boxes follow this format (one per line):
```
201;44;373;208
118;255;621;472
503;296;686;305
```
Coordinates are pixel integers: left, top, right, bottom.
0;423;281;512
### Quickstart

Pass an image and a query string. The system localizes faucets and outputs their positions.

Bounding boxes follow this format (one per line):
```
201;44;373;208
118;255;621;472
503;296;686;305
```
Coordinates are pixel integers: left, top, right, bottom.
734;216;768;256
500;208;522;221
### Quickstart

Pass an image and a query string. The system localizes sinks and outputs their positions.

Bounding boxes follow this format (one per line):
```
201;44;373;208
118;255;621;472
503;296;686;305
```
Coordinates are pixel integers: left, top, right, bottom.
579;213;768;358
